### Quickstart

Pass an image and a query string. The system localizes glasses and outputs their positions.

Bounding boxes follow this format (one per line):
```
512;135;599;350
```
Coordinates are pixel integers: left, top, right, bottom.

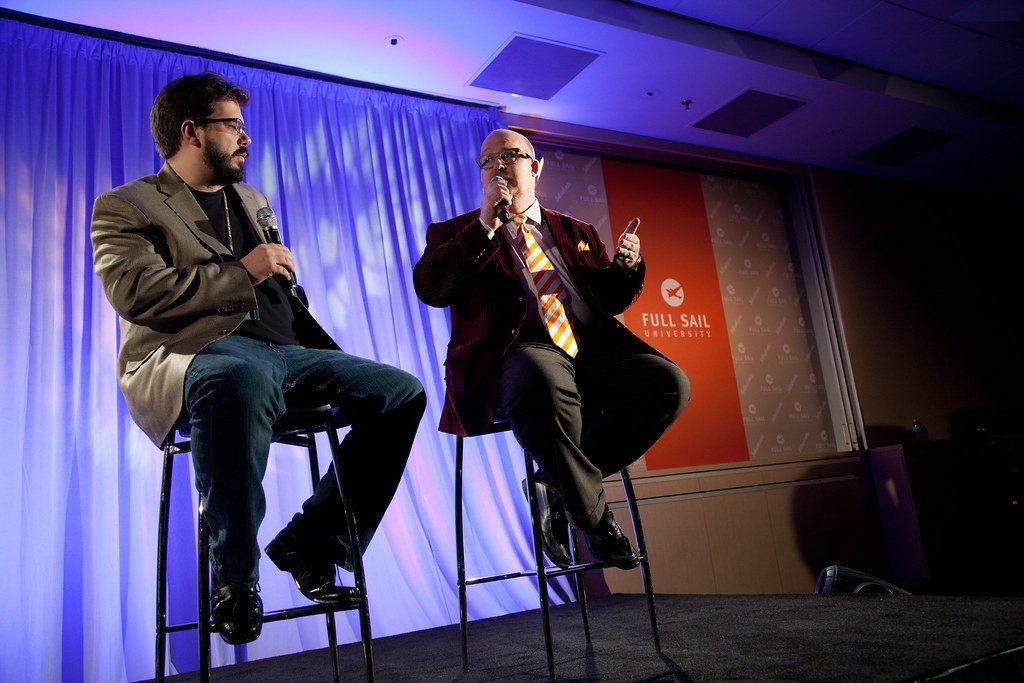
193;118;246;134
475;150;532;169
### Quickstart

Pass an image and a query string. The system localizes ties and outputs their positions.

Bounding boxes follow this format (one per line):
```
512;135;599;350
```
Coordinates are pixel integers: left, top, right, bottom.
510;214;579;358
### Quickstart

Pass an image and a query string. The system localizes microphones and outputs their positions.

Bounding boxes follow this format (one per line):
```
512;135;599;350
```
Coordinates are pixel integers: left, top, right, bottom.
256;207;297;290
491;175;510;223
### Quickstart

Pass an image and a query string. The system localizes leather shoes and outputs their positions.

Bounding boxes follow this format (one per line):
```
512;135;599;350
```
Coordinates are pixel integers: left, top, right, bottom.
522;477;574;569
264;512;362;607
211;566;263;645
583;502;640;570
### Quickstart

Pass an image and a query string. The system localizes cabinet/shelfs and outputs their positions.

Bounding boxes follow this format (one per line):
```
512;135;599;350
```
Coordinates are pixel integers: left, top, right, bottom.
869;436;992;579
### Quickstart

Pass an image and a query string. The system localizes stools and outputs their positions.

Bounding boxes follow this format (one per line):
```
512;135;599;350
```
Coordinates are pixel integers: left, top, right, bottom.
455;408;661;680
155;406;375;683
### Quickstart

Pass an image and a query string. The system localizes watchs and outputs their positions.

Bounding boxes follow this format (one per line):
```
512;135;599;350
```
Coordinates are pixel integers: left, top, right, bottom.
631;255;642;270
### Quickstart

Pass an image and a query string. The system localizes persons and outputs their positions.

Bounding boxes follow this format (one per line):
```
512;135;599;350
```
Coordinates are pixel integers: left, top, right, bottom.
412;130;691;569
90;72;427;645
